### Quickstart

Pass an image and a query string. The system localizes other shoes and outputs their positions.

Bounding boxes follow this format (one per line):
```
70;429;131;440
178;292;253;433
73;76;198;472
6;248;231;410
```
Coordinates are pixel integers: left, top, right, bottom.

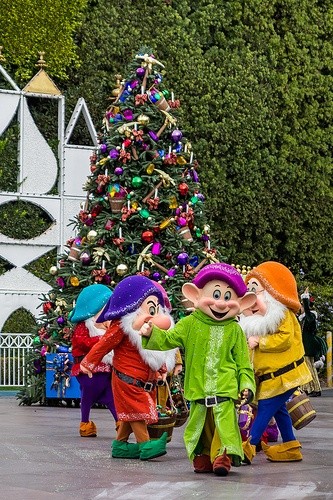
305;390;322;397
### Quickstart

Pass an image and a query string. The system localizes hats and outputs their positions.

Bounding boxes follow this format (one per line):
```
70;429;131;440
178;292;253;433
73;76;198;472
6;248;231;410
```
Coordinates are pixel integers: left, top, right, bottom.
310;296;315;303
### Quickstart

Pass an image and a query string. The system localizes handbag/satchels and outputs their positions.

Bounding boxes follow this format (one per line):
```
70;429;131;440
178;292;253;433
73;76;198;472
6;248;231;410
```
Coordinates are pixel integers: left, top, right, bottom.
314;335;328;360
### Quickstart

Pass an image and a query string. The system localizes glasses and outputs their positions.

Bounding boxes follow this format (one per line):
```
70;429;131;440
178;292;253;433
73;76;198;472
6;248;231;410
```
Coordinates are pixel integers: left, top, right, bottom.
301;303;303;305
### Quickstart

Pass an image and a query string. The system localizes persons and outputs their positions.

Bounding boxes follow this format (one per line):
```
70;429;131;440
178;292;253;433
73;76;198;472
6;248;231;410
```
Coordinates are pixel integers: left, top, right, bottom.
297;286;322;397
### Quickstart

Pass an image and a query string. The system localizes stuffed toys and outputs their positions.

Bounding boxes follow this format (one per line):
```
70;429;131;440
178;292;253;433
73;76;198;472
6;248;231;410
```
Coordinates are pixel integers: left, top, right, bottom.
238;261;314;465
148;277;184;412
80;274;177;460
66;284;123;437
137;264;258;476
